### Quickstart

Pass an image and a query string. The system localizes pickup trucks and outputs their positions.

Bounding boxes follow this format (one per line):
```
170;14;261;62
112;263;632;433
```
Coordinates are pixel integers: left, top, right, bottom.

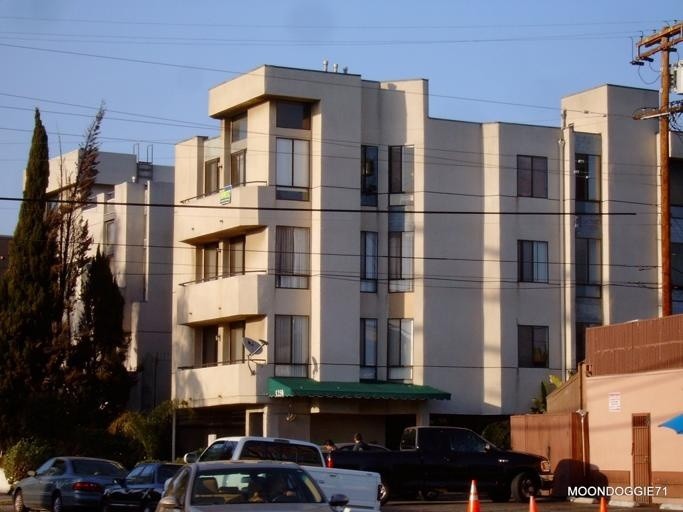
326;426;554;502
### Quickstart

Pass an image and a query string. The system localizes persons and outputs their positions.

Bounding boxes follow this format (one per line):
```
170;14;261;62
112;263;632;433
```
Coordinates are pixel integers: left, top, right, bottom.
323;432;370;451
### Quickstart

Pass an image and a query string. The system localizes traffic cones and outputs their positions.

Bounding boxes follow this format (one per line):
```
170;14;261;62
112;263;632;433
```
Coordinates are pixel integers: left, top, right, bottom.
466;479;481;512
529;495;540;512
599;496;608;512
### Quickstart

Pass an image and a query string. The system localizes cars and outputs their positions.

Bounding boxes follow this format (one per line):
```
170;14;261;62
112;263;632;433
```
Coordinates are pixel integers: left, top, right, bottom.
12;436;391;512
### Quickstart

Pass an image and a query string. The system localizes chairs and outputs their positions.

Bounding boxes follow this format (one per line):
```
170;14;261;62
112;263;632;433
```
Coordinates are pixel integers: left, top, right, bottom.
195;477;219;494
